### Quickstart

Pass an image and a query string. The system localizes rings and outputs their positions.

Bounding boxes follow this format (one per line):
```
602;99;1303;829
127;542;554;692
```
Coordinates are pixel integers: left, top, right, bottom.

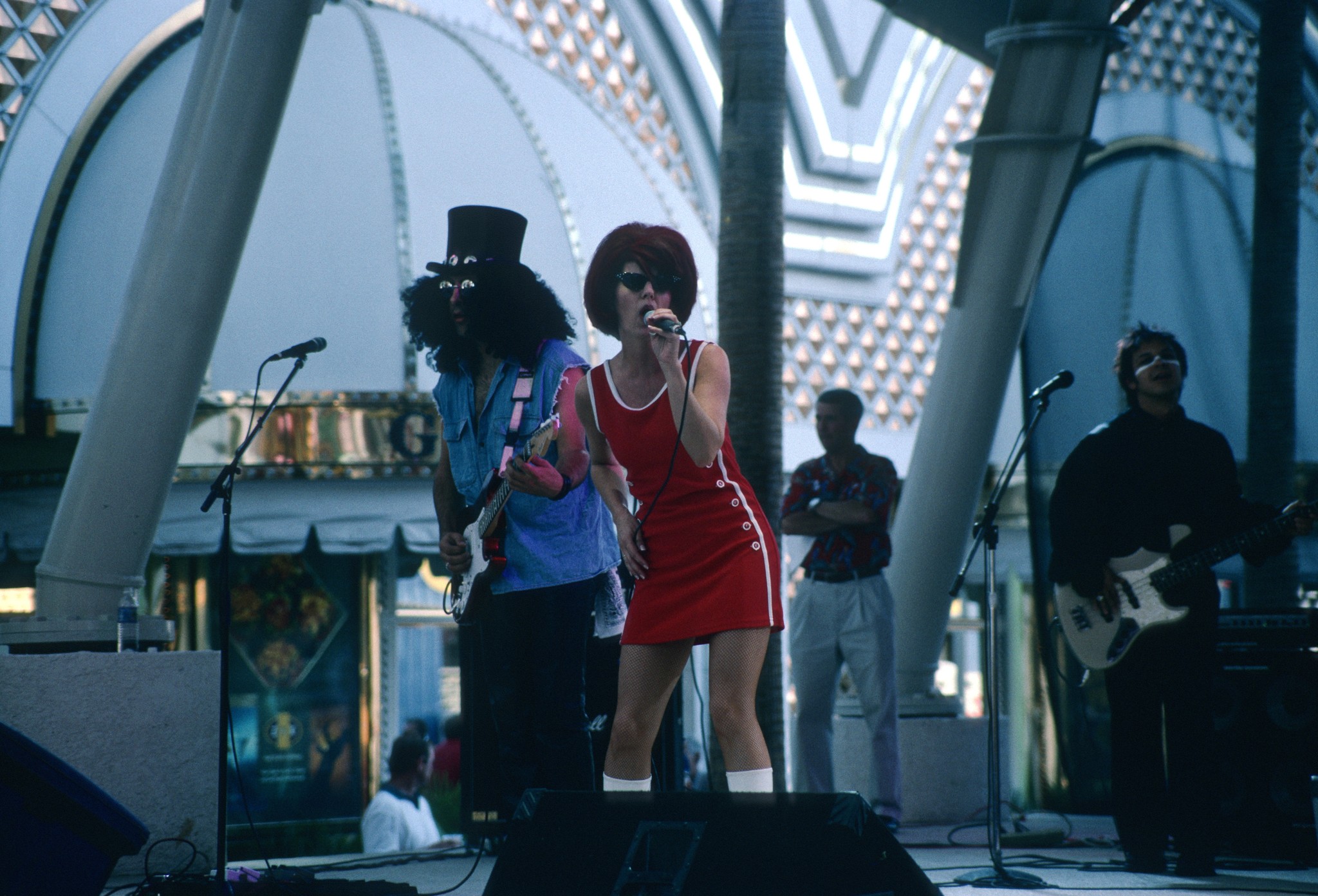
445;562;451;571
1097;596;1104;601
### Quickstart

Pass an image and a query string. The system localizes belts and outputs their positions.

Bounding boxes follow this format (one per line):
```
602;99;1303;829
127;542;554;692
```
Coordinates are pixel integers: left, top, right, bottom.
804;568;865;584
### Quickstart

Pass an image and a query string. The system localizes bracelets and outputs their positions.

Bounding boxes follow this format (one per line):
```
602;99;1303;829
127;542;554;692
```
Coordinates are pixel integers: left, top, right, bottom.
549;473;571;501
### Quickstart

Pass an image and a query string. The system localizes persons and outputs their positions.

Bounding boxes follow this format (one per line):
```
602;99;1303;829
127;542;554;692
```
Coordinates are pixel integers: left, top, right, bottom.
399;204;626;840
782;388;906;849
1048;323;1242;880
572;222;785;795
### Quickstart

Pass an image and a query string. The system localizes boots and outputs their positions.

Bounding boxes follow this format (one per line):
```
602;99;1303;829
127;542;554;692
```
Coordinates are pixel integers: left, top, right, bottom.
725;766;774;792
602;772;652;792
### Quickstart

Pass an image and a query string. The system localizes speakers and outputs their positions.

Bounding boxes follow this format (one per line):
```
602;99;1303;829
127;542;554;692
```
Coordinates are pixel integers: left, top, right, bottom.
490;787;946;895
1099;608;1317;870
0;647;228;896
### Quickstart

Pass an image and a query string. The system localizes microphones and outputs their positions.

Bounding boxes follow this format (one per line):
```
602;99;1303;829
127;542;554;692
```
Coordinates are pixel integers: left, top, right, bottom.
1028;369;1075;400
642;309;681;335
270;337;327;360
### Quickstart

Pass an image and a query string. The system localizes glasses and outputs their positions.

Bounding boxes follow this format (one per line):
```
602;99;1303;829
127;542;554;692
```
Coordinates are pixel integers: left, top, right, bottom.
438;279;478;299
616;271;669;295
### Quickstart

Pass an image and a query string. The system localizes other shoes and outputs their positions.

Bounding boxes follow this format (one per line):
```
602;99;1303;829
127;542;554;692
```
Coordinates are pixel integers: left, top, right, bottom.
876;812;899;834
1174;854;1216;875
1124;850;1164;874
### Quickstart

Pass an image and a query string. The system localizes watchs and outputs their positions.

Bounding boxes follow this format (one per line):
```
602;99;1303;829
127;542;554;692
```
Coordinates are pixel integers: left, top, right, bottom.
809;497;825;517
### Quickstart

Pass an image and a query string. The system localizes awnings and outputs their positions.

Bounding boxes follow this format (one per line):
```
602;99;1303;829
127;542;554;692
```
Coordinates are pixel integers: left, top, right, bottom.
0;482;482;556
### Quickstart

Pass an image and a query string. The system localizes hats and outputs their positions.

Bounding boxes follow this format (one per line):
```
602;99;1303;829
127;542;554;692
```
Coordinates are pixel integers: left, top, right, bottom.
426;204;528;275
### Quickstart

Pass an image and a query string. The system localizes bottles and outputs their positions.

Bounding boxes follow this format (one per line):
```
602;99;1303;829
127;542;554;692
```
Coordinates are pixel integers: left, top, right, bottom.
117;589;139;654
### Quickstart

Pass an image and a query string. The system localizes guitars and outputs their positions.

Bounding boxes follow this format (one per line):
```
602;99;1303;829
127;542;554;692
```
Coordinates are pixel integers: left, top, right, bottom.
449;415;550;620
1051;489;1312;675
